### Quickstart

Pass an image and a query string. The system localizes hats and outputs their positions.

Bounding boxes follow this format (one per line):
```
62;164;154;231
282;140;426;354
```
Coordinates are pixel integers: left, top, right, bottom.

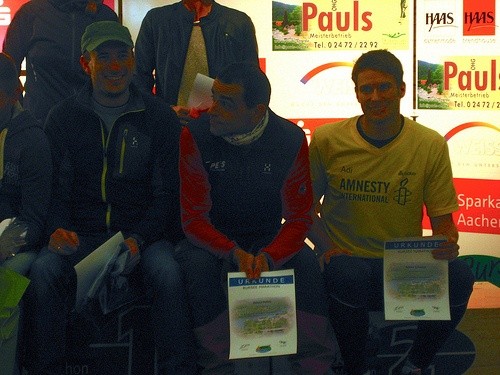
81;21;134;52
0;52;17;77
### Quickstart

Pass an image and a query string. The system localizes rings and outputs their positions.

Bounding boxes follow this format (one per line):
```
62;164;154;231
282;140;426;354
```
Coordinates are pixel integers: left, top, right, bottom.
57;246;60;250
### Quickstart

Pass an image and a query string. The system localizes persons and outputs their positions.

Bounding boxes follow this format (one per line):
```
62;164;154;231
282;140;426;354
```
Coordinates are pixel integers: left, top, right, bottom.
304;49;473;375
0;0;313;375
